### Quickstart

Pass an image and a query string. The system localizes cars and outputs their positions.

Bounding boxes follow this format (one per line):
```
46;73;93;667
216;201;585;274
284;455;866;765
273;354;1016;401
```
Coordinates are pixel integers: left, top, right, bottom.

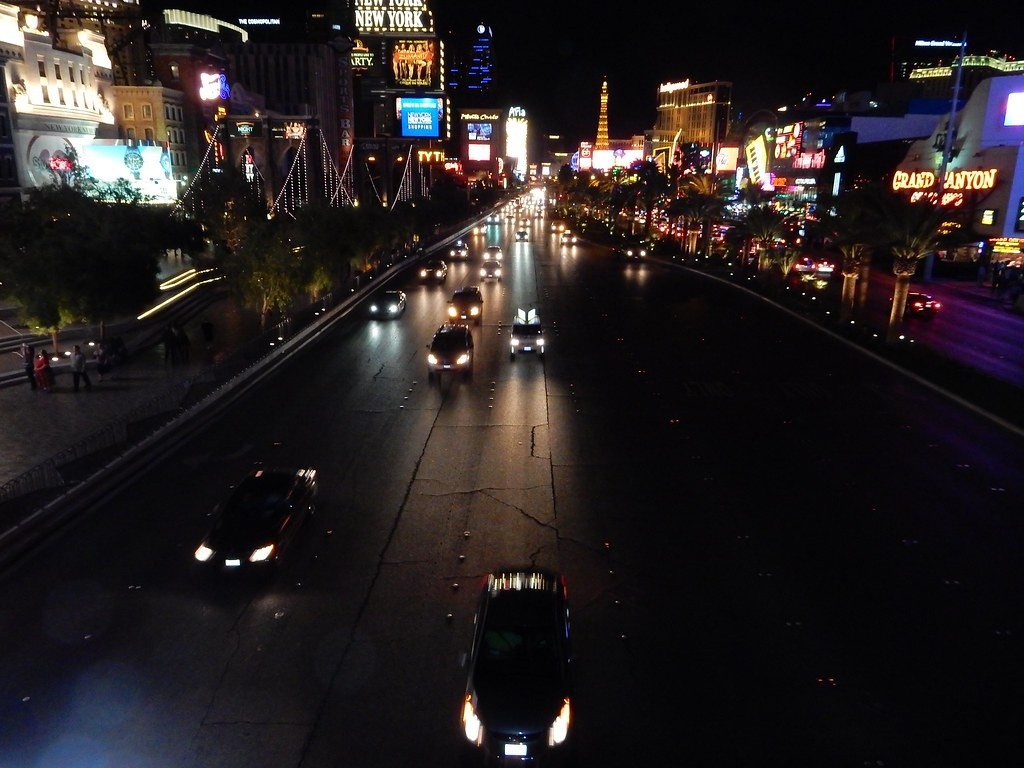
192;461;318;590
639;209;758;261
484;190;544;224
420;261;447;284
449;243;469;259
369;291;408;319
480;245;503;283
888;292;941;318
612;242;643;259
459;563;573;768
551;220;576;245
793;256;834;273
515;232;529;242
474;225;486;234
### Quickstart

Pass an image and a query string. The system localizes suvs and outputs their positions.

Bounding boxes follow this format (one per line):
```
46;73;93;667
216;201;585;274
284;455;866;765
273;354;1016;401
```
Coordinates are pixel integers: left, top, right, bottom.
447;286;483;325
510;315;545;359
427;321;473;378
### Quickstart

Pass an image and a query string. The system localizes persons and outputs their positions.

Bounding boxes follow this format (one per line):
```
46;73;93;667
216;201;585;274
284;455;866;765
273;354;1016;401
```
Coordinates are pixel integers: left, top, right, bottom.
393;43;431;80
162;324;191;368
33;349;56;390
11;342;36;391
93;342;107;383
108;336;128;380
69;345;90;392
